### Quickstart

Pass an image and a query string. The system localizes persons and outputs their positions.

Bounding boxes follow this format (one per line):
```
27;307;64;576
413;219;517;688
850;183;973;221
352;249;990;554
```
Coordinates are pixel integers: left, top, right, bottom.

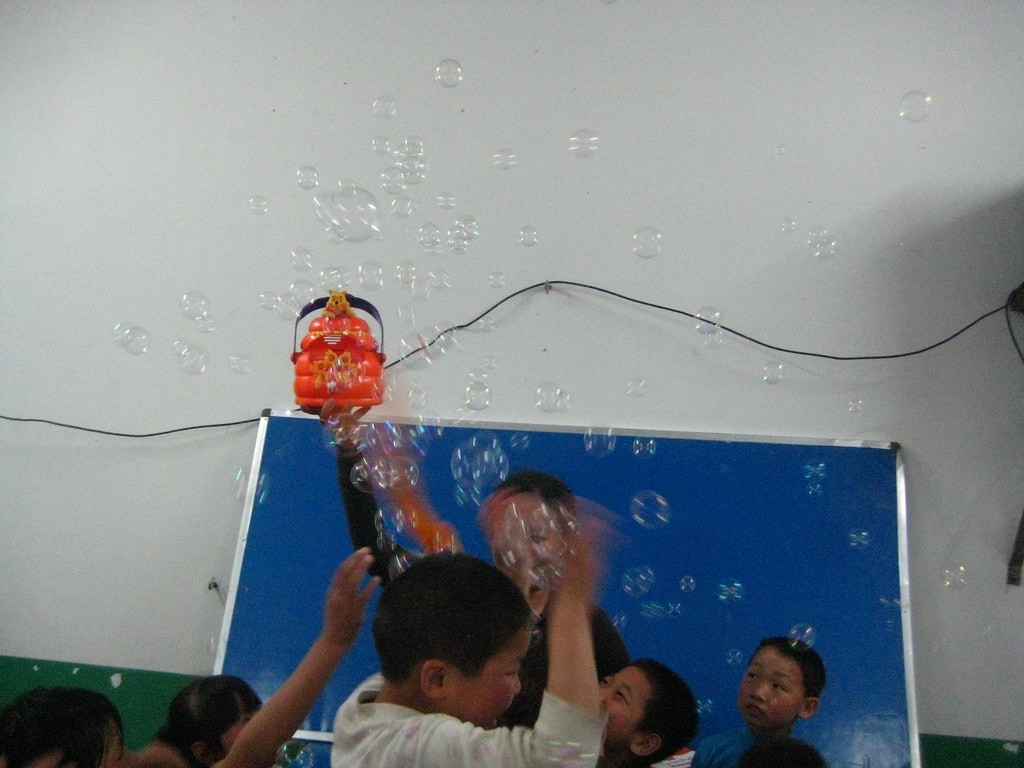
0;547;380;768
301;397;829;768
